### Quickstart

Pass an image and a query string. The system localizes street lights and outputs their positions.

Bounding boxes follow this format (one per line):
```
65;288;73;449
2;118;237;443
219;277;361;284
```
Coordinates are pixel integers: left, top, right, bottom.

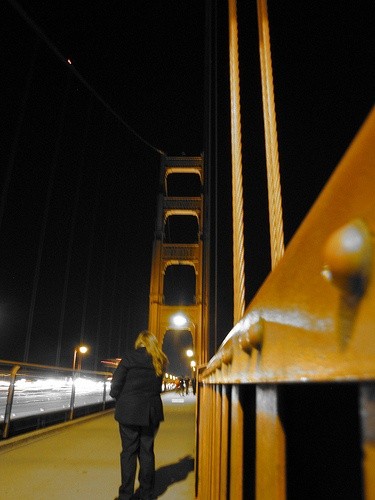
73;346;88;370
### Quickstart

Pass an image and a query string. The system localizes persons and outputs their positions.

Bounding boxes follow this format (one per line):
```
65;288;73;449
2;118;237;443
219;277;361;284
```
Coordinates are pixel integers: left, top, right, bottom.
175;377;196;395
110;331;170;500
